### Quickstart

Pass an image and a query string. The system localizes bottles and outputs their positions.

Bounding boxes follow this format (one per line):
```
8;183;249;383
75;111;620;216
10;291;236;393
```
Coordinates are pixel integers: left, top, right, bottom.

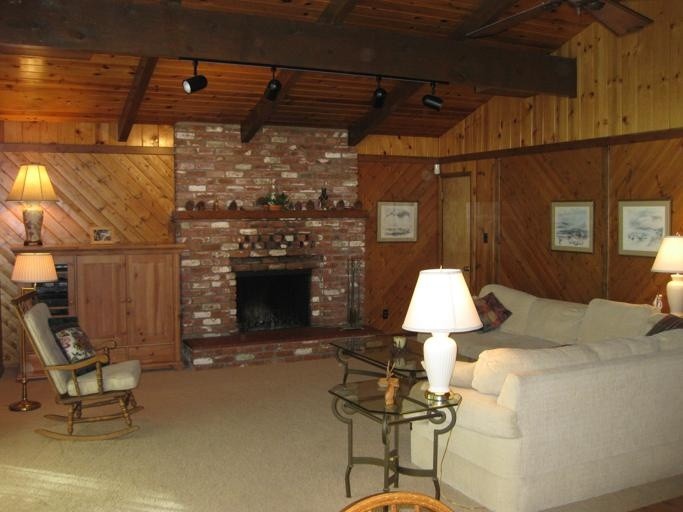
320;188;329;211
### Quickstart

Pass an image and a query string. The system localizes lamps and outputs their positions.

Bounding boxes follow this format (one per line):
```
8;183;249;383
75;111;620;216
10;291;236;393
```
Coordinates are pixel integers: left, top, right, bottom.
403;266;483;401
4;163;60;247
178;56;450;112
651;233;682;319
8;253;60;413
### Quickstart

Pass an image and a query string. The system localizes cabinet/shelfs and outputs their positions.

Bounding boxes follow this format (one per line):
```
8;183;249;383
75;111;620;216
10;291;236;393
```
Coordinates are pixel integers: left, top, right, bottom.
74;248;184;373
11;248;76;381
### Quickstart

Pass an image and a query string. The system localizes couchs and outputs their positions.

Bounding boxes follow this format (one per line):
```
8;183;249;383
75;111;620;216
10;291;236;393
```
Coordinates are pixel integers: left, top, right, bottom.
407;284;682;512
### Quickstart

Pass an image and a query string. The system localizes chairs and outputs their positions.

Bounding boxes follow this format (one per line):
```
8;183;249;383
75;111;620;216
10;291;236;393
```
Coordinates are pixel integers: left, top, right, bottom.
12;291;144;442
340;492;452;512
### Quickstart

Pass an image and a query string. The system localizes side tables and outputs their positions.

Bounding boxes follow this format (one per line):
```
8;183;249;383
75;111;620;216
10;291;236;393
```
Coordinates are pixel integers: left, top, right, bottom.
329;379;461;499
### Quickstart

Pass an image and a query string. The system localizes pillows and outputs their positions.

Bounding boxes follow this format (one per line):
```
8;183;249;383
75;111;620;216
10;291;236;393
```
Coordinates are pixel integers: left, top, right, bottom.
472;284;683;395
421;360;478;390
48;316;106;377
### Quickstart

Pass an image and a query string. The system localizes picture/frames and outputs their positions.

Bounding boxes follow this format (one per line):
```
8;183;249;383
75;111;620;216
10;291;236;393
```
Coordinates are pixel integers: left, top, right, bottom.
376;202;418;242
618;200;670;258
89;227;116;245
551;202;594;253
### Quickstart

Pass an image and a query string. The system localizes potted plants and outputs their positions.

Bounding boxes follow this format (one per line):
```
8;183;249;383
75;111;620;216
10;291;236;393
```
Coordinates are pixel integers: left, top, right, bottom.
256;193;288;213
